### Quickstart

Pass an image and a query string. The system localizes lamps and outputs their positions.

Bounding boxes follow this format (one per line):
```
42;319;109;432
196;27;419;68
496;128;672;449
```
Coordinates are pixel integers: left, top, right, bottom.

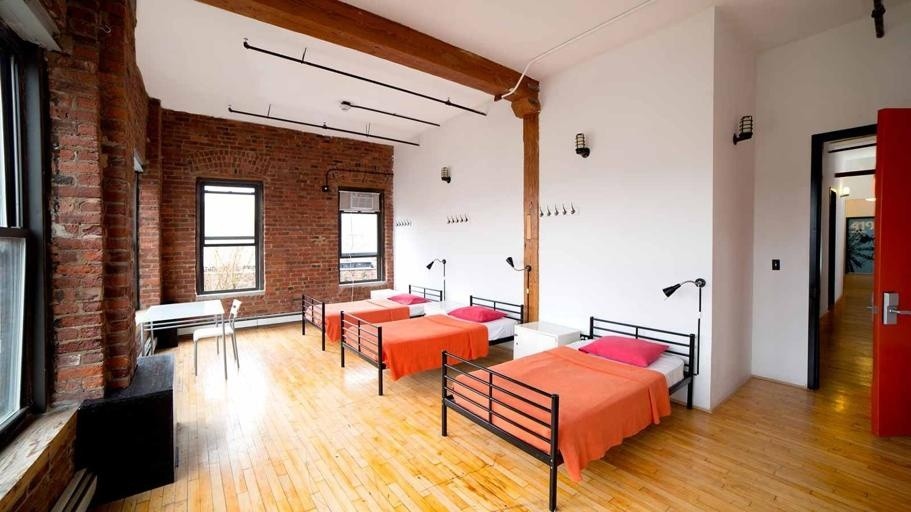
506;257;532;272
656;278;706;301
337;101;352;112
734;114;753;143
575;133;589;157
425;259;446;270
440;167;451;183
839;187;850;197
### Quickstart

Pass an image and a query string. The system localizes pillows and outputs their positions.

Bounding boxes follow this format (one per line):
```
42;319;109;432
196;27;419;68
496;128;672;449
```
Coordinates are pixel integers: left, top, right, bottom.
387;293;432;306
578;335;669;367
448;306;508;323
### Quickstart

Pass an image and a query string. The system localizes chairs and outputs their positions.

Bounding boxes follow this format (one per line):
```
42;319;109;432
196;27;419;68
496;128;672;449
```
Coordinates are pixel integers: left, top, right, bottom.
193;298;242;376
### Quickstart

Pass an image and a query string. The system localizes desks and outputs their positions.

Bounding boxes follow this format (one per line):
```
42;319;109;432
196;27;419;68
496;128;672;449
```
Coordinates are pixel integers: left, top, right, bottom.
139;298;228;381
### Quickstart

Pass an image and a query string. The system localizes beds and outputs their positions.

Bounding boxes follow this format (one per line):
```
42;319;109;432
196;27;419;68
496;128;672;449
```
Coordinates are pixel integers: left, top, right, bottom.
340;294;523;397
440;316;695;511
302;284;443;351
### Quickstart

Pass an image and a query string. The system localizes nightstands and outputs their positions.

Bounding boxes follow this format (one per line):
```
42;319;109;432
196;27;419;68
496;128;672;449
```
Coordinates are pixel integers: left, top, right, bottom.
423;300;468;315
513;320;580;359
